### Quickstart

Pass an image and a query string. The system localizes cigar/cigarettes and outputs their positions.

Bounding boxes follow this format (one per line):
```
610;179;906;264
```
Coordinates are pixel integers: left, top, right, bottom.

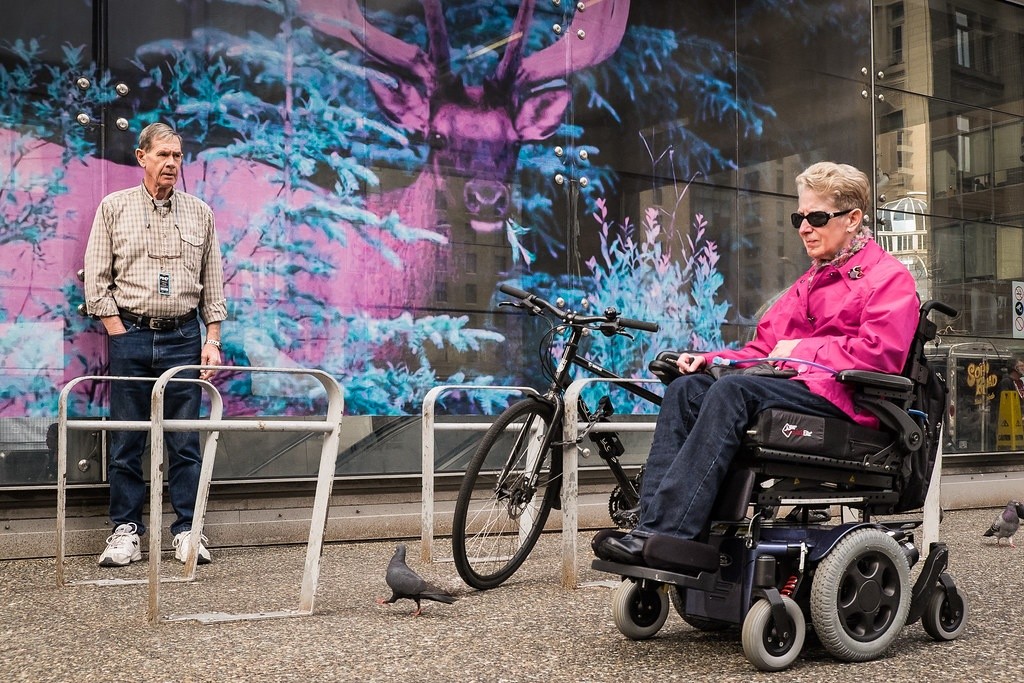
202;373;211;383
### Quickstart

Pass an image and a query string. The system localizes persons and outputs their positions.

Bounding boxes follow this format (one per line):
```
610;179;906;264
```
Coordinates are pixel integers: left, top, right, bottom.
592;160;921;566
83;122;228;566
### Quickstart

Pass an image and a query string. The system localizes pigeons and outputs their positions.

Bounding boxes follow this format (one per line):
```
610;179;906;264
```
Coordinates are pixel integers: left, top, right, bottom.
375;542;460;616
981;498;1020;550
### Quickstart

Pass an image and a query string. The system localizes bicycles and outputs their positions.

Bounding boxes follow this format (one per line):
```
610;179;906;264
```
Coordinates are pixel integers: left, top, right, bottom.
450;284;712;591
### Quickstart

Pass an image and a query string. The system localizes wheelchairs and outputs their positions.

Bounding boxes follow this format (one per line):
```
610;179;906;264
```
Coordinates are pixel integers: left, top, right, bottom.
611;299;970;672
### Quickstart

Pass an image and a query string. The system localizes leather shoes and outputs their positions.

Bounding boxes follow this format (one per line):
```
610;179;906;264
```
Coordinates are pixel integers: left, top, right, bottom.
598;534;651;566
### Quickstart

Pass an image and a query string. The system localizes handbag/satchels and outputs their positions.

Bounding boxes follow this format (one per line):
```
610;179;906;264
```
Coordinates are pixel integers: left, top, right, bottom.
708;361;797;381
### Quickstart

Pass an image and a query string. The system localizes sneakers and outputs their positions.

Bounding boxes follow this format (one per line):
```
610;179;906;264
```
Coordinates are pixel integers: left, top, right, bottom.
98;522;142;567
172;530;211;564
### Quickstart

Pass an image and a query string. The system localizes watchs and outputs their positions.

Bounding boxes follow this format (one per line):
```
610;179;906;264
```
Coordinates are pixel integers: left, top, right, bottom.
204;340;222;351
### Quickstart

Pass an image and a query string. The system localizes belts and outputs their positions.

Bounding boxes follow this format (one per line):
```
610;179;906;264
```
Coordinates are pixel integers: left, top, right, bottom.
118;309;197;330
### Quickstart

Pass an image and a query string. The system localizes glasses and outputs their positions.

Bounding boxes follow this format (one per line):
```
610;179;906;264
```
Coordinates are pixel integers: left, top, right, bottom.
791;209;852;229
146;223;183;260
